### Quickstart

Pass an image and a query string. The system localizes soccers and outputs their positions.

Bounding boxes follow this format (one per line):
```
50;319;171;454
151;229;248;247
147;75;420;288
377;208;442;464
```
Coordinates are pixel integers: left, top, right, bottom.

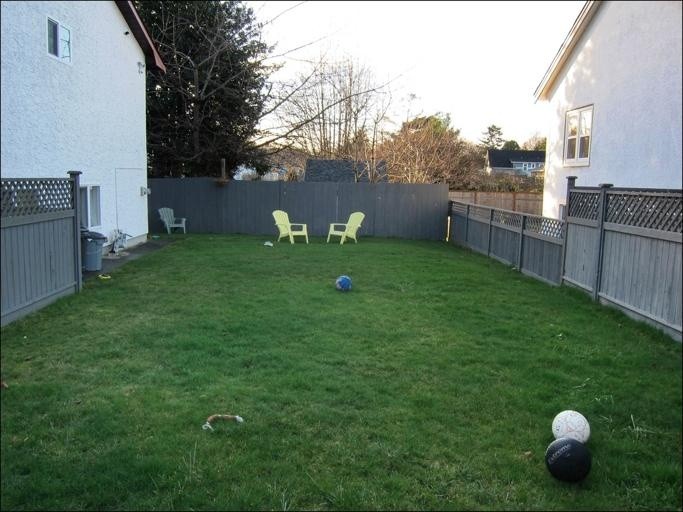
552;410;590;443
545;438;592;482
335;276;352;290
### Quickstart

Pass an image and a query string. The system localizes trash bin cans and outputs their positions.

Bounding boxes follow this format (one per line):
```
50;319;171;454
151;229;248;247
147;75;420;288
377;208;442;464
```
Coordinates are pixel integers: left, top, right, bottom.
81;232;108;272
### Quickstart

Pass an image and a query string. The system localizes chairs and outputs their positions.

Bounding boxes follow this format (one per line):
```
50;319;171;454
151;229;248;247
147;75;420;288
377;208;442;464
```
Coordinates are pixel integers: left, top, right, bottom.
325;211;366;245
157;206;186;234
271;210;308;245
